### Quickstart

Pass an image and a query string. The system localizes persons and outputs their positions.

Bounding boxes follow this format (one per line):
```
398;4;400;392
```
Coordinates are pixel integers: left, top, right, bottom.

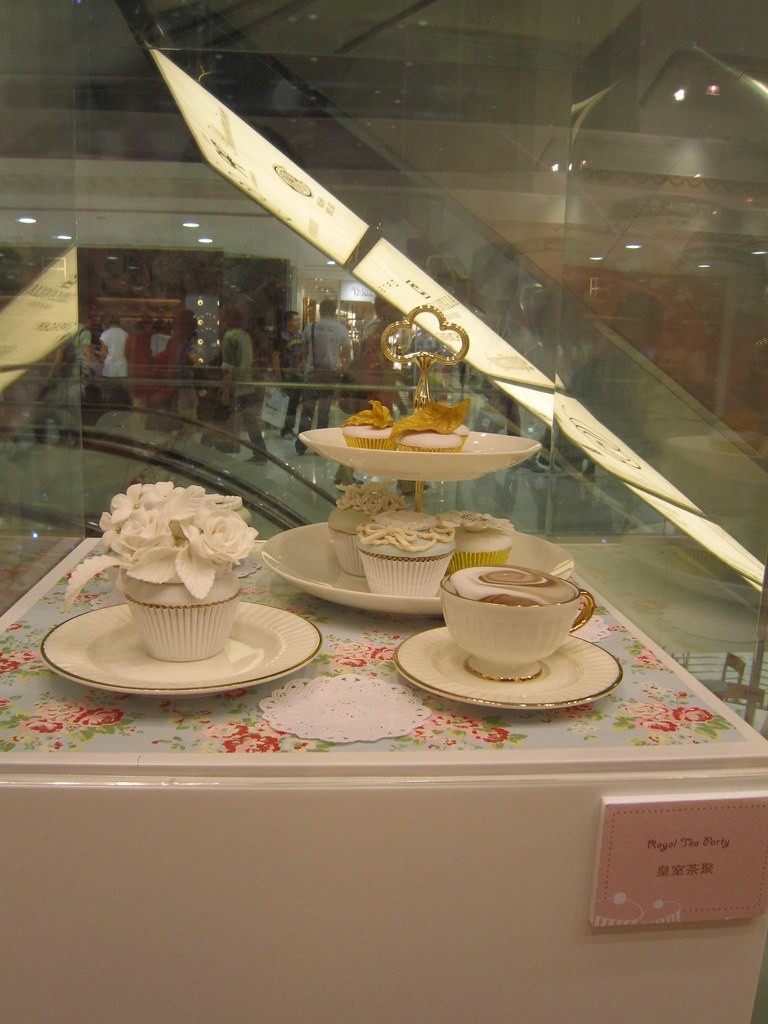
37;296;435;495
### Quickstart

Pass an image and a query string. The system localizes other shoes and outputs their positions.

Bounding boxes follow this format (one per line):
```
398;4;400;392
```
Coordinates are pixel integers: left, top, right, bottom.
245;456;267;466
215;442;240;454
281;429;293;441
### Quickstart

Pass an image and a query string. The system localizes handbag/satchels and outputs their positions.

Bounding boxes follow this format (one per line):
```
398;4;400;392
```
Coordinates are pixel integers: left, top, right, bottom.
339;357;384;415
306;364;337;398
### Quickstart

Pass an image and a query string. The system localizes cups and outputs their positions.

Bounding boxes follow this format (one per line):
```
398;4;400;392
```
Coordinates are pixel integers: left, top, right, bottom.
439;575;597;683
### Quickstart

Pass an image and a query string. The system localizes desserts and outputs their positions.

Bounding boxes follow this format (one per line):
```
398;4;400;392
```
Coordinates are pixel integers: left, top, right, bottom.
325;482;515;599
340;398;472;454
62;480;259;662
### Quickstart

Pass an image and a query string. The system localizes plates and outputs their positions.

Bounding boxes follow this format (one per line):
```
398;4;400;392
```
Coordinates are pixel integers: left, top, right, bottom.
38;600;323;697
392;625;624;711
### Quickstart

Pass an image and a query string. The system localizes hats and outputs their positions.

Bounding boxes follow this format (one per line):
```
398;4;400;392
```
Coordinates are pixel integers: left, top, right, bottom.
135;312;162;330
